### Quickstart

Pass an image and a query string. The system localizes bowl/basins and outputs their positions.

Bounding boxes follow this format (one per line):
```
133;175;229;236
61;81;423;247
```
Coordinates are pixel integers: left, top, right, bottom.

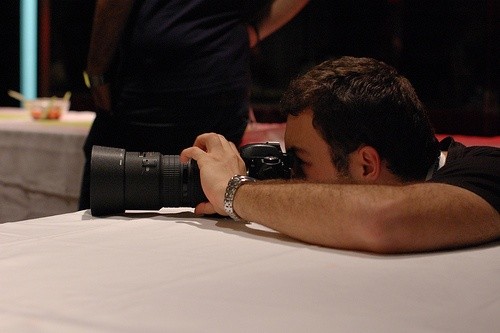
24;97;70;120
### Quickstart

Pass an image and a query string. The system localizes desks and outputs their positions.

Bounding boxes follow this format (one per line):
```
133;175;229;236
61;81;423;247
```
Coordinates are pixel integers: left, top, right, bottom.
1;106;98;225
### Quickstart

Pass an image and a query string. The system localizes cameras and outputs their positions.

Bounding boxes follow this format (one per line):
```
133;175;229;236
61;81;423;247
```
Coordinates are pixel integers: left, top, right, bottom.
90;141;289;214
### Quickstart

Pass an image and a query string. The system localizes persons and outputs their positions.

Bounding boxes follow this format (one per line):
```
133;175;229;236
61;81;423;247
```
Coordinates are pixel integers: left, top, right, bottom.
93;1;420;151
178;56;500;254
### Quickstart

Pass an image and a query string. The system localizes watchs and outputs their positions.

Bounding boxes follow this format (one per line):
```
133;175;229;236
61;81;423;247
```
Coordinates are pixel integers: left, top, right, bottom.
224;175;257;224
